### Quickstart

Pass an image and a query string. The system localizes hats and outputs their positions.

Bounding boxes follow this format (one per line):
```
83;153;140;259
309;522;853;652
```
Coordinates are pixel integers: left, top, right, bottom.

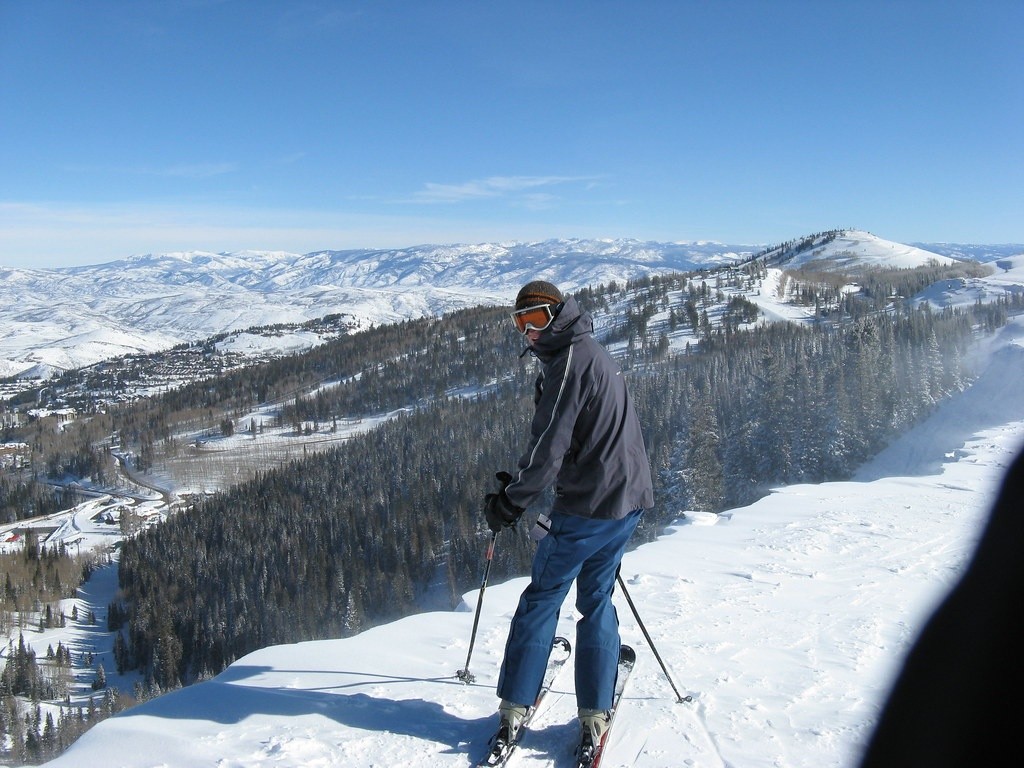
515;281;563;310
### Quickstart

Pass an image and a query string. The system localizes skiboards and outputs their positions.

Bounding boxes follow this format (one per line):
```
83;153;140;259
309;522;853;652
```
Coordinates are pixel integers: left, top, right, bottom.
474;638;637;768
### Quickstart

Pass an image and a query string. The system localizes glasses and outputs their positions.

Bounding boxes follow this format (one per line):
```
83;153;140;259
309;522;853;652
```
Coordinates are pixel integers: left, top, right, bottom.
509;302;565;335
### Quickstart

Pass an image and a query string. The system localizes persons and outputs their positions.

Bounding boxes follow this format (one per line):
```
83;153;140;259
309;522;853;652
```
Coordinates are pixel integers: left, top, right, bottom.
483;281;655;743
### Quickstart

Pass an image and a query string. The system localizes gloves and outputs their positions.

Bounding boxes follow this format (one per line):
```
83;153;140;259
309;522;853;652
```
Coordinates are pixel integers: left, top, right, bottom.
483;472;525;534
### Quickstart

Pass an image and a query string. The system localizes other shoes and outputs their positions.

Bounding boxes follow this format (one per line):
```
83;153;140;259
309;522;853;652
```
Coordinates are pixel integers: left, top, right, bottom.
576;706;613;737
498;698;532;725
